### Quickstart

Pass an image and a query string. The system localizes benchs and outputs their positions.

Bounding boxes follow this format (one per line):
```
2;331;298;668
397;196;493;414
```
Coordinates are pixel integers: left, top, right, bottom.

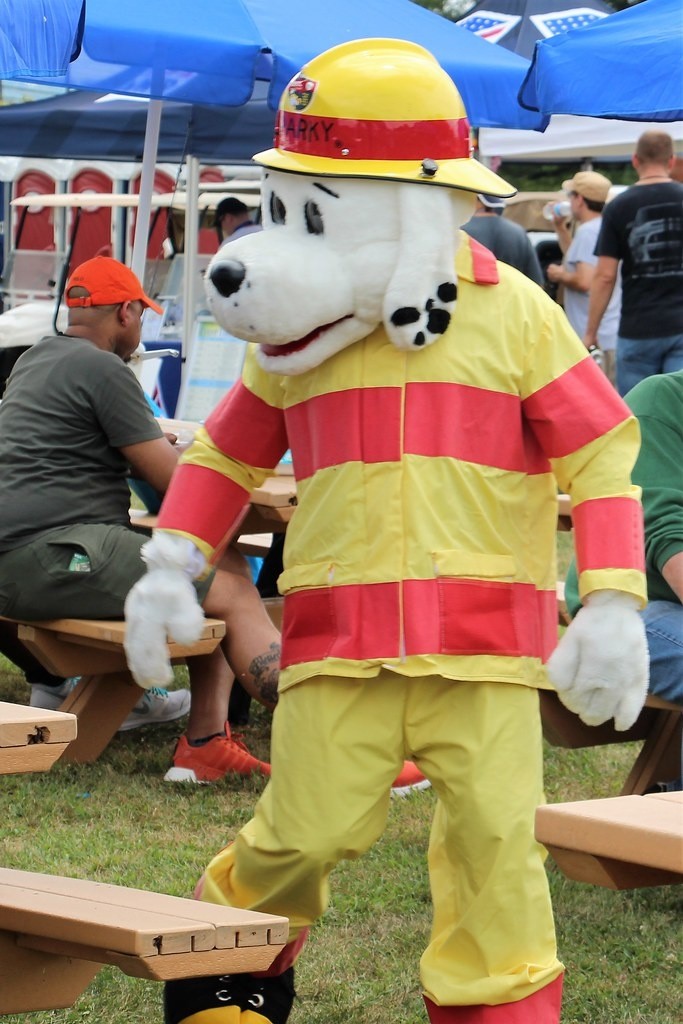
537;684;683;798
0;614;225;763
0;867;289;1016
532;791;683;890
127;508;275;558
0;701;79;775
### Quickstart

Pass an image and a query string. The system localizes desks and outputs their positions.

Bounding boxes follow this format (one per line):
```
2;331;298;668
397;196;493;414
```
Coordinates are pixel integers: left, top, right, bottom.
127;441;299;535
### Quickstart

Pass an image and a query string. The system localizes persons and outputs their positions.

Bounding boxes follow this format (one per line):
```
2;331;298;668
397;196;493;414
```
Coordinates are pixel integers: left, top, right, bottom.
582;130;683;398
460;193;543;287
0;256;431;797
215;201;262;248
565;368;682;797
547;171;622;391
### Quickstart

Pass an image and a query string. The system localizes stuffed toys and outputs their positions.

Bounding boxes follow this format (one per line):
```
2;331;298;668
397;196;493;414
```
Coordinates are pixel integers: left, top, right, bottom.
124;37;651;1023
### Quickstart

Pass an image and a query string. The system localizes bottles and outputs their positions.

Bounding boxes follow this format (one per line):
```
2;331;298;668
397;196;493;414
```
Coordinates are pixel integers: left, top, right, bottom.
541;200;572;222
590;344;605;371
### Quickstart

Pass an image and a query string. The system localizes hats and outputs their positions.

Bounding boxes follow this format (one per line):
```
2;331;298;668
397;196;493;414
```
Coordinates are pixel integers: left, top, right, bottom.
65;256;163;315
208;197;250;229
477;192;506;208
562;171;612;202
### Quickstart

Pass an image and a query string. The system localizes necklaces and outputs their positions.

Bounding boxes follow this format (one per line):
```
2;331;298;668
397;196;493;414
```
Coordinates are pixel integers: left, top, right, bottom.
641;175;668;178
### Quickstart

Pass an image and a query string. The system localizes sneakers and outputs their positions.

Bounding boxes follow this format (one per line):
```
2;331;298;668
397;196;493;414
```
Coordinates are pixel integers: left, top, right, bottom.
388;760;433;798
29;676;84;712
115;686;191;729
163;721;272;790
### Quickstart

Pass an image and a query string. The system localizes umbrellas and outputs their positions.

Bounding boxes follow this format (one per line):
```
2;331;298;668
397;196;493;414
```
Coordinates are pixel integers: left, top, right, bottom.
0;91;275;166
1;0;551;134
454;0;616;60
518;0;683;122
